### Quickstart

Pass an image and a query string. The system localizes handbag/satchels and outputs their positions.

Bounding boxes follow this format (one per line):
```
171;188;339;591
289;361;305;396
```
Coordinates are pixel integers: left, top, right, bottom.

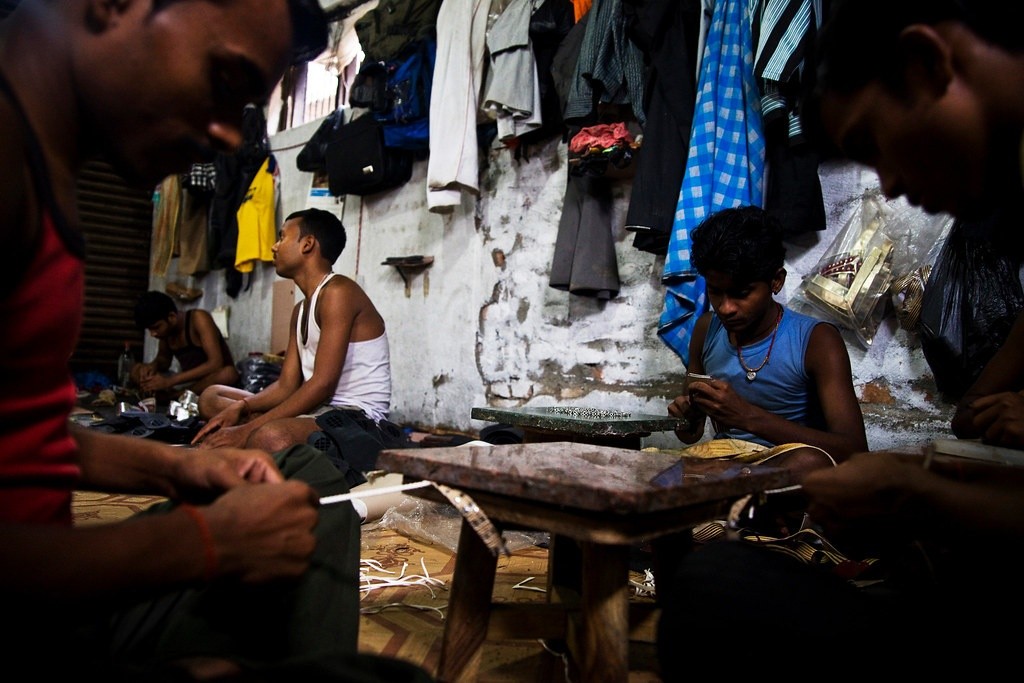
896;211;1024;402
793;193;899;351
651;524;895;683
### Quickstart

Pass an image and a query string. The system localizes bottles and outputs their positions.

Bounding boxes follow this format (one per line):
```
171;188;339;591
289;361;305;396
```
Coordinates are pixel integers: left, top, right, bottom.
118;341;138;388
240;349;272;391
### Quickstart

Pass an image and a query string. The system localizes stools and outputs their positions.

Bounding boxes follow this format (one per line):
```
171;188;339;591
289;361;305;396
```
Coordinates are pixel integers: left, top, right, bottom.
374;440;791;683
466;407;693;449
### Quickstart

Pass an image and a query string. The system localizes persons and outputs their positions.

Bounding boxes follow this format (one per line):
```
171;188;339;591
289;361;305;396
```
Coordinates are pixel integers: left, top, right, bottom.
190;208;390;496
0;1;432;683
537;207;869;652
131;291;240;405
799;0;1024;534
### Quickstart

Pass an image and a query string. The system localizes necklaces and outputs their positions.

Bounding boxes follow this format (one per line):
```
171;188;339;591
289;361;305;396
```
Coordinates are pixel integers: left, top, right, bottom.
736;306;781;381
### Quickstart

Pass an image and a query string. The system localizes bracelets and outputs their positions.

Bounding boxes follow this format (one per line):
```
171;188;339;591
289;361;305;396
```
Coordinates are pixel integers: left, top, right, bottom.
242;398;252;410
185;506;216;573
